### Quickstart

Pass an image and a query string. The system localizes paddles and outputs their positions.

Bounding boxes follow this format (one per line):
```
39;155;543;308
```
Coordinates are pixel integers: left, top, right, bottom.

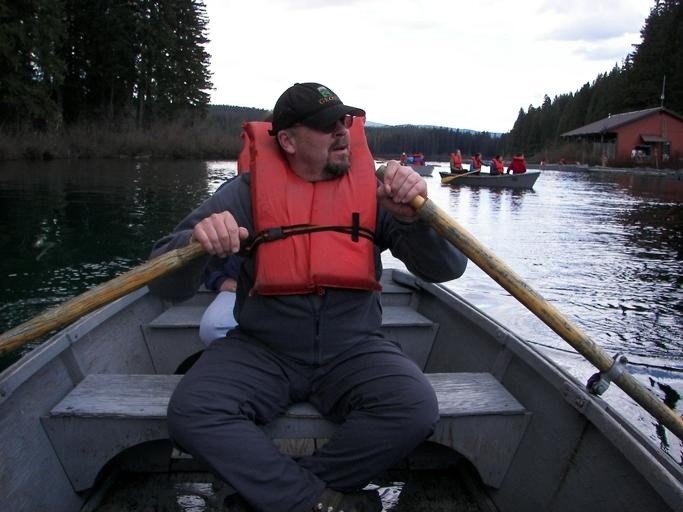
441;169;481;182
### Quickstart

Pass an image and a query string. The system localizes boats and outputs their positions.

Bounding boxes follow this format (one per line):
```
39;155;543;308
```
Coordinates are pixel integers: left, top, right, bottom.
438;171;541;191
539;164;589;172
396;163;435;177
0;259;683;512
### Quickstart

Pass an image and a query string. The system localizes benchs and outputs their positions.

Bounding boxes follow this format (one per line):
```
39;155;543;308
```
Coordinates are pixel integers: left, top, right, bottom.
144;302;439;374
41;371;531;497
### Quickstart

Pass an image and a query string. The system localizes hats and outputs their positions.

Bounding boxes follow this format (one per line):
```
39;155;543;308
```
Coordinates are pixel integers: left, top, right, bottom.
267;82;365;136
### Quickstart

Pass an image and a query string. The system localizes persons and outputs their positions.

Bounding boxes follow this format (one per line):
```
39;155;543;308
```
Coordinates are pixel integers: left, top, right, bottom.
470;153;490;176
490;155;504;176
146;82;470;512
199;248;245;349
400;151;426;166
506;152;526;175
448;149;468;174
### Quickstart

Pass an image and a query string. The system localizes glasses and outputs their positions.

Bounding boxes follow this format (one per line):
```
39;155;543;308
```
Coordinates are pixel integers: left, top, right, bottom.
320;114;354;134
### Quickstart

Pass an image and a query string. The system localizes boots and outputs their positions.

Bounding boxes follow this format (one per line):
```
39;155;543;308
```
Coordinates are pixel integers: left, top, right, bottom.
311;487;383;512
